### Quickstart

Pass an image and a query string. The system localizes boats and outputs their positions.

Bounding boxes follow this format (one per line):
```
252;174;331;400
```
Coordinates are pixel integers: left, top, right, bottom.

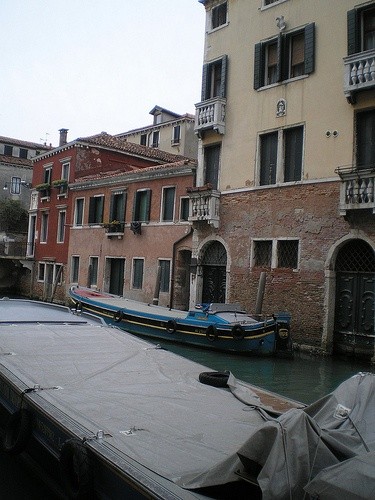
68;281;292;359
0;297;375;500
